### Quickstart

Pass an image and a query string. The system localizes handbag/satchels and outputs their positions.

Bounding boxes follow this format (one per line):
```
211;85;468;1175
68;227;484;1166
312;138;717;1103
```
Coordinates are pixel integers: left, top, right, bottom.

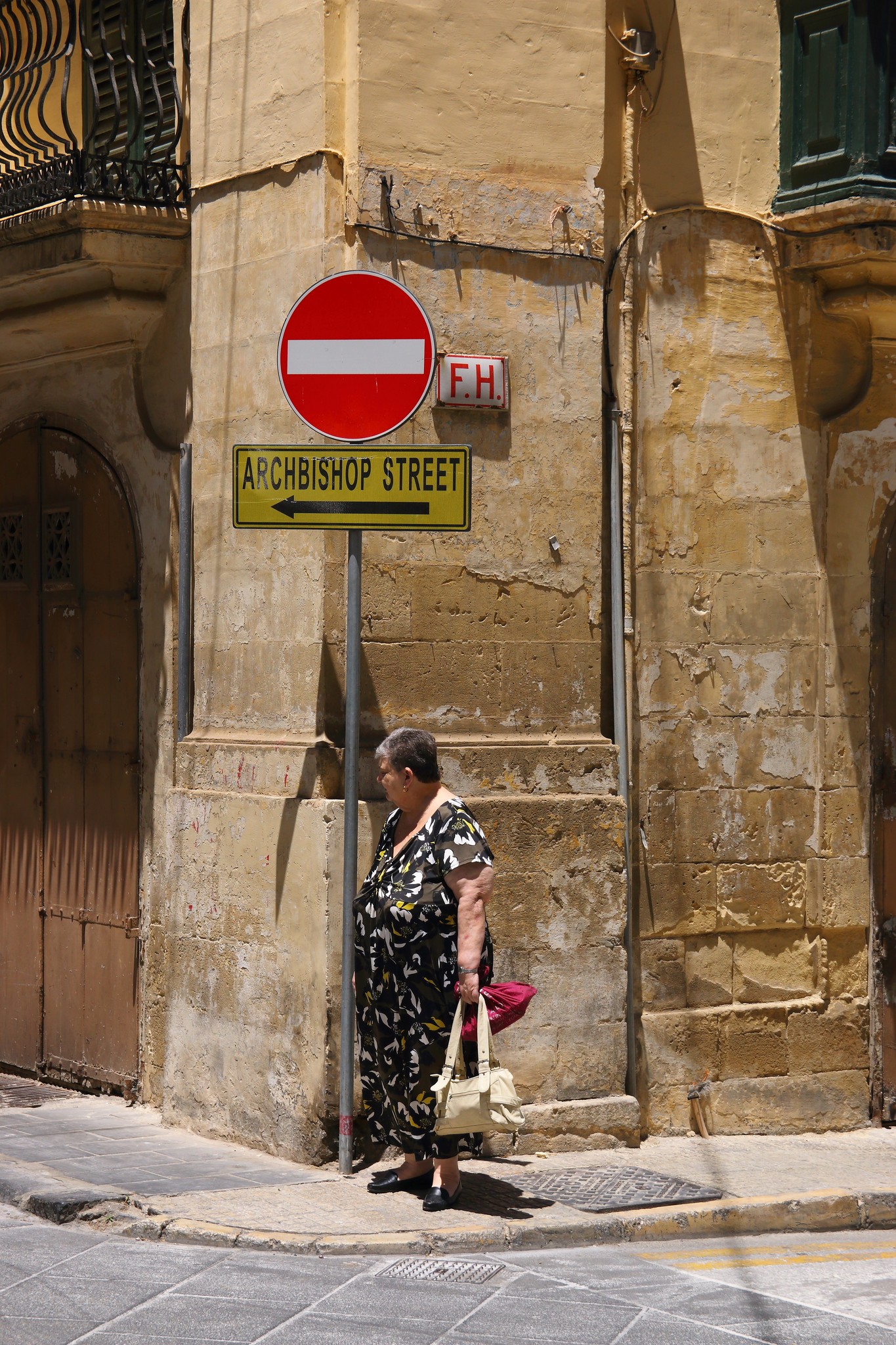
429;993;526;1136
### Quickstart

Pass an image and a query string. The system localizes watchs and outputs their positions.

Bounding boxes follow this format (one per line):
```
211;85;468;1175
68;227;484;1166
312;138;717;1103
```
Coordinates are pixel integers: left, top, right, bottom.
457;966;477;974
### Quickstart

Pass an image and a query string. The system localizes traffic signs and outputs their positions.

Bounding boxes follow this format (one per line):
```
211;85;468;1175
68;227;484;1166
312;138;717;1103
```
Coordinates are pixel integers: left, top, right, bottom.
231;441;471;530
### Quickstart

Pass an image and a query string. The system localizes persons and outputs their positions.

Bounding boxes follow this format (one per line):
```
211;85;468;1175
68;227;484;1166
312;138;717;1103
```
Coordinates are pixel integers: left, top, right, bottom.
347;729;496;1210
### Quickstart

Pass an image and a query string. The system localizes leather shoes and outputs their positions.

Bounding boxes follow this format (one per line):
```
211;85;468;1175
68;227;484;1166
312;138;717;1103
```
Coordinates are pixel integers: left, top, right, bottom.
368;1167;436;1194
423;1179;463;1210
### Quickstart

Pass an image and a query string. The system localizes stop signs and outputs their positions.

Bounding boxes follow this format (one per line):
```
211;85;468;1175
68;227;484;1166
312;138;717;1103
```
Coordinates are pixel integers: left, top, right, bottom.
275;269;435;443
